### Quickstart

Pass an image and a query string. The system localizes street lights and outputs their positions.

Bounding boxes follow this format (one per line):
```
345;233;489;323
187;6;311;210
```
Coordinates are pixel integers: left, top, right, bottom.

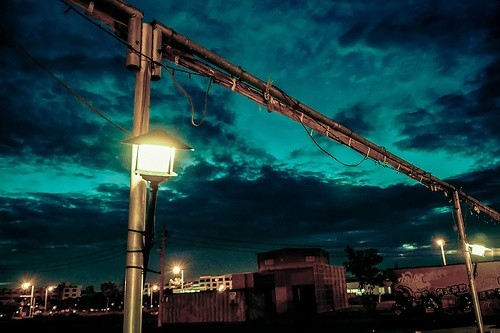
436;238;447;265
150;284;157;308
21;283;34;317
125;124;196;333
44;284;54;311
173;266;184;290
